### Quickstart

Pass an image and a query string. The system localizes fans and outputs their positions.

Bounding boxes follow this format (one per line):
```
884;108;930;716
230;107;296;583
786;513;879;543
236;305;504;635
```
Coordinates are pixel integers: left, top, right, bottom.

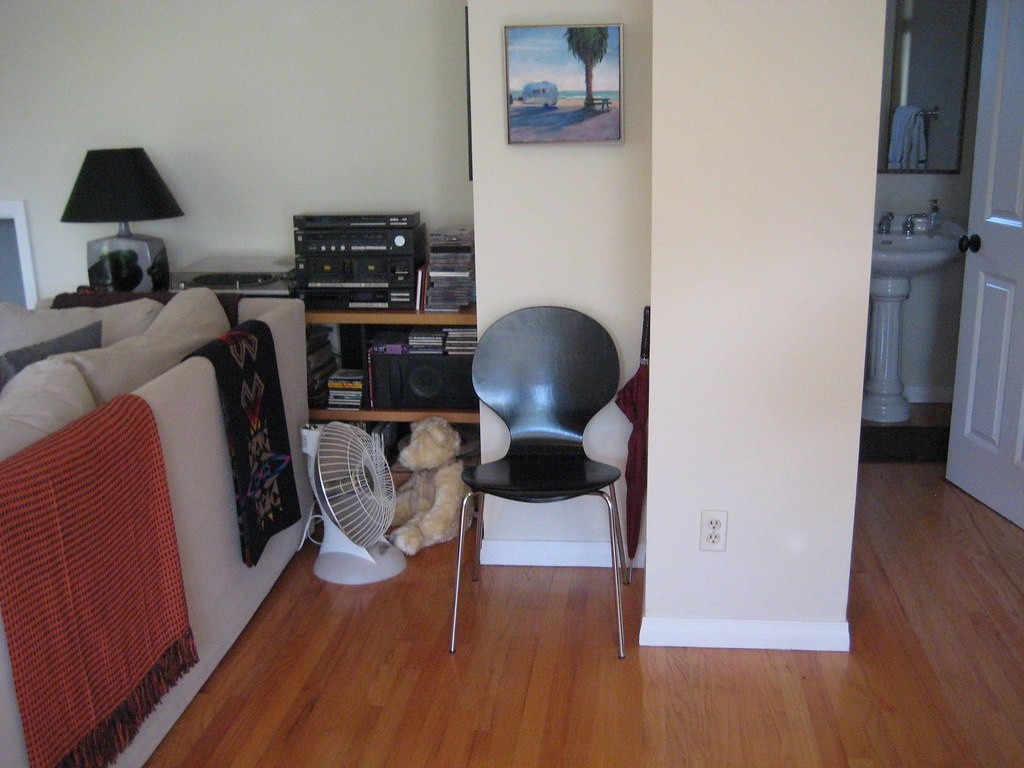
299;421;409;586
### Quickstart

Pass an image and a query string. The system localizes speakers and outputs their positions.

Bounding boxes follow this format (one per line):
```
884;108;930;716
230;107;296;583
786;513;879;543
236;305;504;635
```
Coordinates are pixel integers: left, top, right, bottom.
369;350;480;414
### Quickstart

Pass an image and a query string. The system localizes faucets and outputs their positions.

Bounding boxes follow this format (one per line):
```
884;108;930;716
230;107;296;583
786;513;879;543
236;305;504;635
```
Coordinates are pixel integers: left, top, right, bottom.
877;212;894;235
902;213;928;237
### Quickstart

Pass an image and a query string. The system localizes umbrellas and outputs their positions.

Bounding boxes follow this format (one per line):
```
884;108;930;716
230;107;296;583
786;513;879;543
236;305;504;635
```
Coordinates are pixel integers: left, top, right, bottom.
614;305;651;587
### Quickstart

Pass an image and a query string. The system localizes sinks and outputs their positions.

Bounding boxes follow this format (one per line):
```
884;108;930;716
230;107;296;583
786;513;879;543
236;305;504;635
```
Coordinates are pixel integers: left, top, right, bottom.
871;215;966;277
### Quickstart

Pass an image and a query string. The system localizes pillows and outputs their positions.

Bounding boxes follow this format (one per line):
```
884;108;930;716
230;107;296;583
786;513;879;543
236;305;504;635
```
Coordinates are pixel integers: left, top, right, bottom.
1;285;224;465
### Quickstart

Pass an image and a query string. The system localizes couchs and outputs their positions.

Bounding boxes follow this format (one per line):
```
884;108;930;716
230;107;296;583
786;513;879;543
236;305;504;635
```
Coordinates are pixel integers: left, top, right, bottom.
0;296;316;768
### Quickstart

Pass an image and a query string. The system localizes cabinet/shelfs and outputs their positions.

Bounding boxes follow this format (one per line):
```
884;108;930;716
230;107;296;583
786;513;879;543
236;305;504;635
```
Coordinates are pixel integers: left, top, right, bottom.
304;308;480;455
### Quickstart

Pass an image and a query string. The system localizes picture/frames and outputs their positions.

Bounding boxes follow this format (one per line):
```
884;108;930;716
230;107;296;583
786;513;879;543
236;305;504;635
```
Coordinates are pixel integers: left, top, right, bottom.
502;22;626;147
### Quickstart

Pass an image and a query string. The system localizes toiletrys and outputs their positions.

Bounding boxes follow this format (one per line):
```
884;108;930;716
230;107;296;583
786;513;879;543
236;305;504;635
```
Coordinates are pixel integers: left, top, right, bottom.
928;199;943;236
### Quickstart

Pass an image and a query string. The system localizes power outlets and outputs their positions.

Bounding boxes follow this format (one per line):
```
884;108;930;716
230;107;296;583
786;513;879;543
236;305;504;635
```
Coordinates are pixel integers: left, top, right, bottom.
700;509;728;552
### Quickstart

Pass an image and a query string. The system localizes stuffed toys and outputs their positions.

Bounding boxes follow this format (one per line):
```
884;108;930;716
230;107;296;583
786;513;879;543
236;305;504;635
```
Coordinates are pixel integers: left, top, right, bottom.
382;415;473;556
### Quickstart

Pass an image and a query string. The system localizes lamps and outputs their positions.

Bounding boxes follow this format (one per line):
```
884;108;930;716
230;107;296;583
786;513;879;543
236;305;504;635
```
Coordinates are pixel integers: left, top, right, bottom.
59;147;184;293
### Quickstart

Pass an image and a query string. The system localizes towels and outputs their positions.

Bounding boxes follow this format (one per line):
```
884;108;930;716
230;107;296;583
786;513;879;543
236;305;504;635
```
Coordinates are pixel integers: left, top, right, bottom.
887;105;926;170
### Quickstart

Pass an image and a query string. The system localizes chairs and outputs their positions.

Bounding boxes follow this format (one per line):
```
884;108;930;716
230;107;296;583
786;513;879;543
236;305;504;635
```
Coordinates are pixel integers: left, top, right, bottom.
450;305;631;660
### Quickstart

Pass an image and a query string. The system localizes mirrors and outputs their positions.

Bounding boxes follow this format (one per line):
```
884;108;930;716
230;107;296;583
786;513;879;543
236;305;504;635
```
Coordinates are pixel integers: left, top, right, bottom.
877;0;975;176
